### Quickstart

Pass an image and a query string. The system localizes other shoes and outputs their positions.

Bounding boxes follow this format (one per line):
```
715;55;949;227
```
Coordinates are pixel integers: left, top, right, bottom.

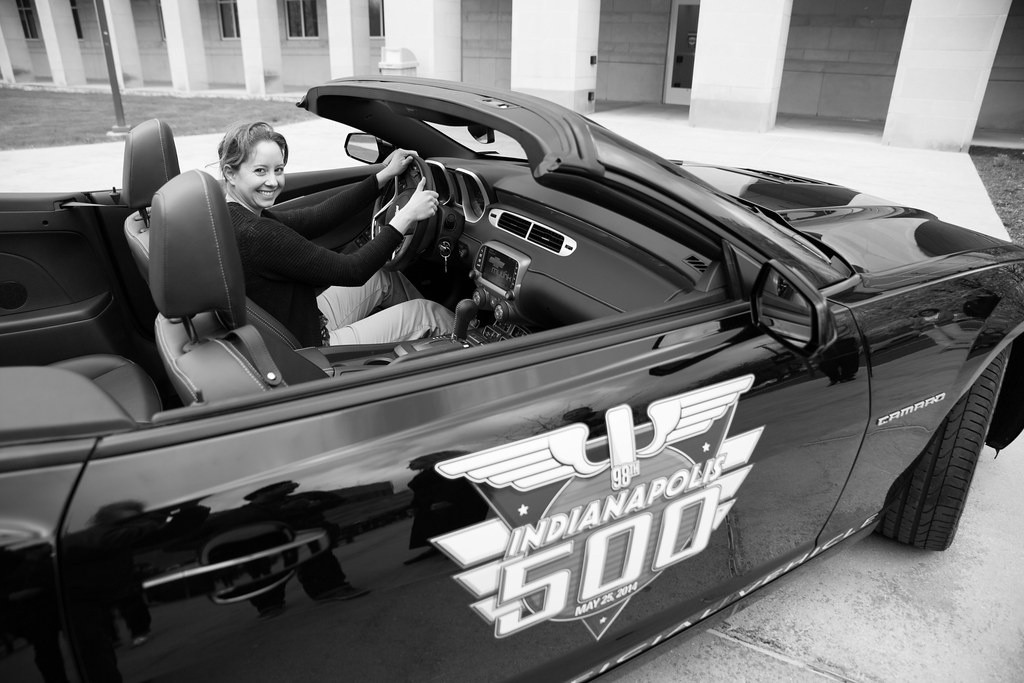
260;600;283;617
130;630;152;648
314;582;370;603
404;546;440;565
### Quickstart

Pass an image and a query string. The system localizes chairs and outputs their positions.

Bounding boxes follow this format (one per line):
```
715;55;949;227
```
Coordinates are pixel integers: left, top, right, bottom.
122;116;300;348
44;354;163;423
150;172;280;404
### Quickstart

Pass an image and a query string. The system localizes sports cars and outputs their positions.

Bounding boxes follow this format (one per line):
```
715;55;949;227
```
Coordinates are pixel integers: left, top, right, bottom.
0;71;1024;683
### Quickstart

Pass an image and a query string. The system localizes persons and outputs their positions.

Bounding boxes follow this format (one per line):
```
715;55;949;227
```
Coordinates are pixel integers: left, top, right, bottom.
217;122;481;347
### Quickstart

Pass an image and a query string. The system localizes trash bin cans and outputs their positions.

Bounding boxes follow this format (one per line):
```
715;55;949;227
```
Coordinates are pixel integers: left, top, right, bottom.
379;45;420;77
